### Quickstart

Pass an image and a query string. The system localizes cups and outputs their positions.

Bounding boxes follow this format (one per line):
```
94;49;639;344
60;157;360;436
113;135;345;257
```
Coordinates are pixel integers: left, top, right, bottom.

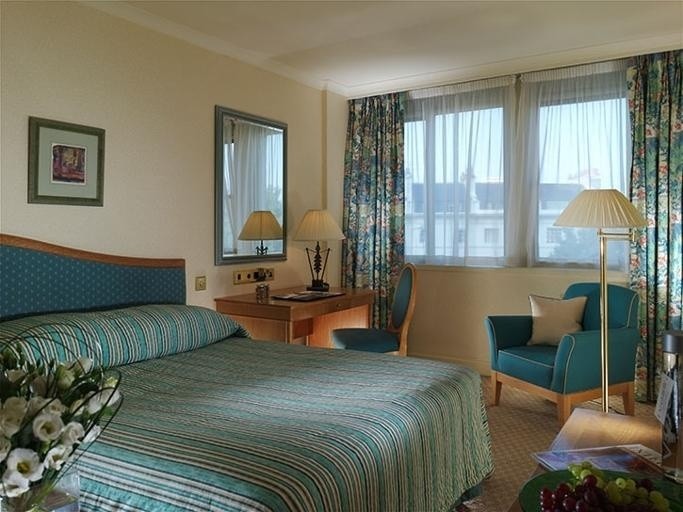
662;330;683;467
256;284;270;300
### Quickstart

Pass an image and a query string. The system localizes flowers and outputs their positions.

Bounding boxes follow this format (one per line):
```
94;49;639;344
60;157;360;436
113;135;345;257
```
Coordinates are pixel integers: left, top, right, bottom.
0;323;125;512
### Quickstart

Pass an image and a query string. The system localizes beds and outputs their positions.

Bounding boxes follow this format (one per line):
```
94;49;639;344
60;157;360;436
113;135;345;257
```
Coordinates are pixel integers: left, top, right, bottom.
0;233;493;512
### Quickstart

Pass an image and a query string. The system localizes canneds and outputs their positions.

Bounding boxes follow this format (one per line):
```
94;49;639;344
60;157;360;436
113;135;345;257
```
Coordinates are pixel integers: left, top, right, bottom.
256;284;269;304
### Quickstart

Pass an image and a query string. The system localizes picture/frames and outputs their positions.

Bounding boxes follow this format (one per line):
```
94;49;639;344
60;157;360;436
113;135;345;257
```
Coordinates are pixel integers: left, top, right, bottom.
27;117;105;207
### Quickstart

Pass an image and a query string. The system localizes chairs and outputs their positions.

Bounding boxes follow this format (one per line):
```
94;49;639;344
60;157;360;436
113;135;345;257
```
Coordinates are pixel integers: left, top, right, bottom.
482;282;640;429
330;263;417;357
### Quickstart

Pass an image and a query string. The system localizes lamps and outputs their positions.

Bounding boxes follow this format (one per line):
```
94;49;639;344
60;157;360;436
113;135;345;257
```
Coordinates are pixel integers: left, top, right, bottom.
237;210;284;256
553;189;648;415
292;209;346;292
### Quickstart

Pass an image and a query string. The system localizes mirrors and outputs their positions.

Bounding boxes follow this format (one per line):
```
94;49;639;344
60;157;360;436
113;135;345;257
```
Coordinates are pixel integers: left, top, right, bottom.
214;104;288;266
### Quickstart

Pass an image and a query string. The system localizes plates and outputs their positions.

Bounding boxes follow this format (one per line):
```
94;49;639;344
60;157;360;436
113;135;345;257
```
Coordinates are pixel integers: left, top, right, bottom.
522;467;683;509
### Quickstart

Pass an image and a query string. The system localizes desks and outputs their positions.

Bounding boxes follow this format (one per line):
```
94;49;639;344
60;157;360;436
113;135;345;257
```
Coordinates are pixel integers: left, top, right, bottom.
214;286;375;349
660;330;683;445
508;408;682;512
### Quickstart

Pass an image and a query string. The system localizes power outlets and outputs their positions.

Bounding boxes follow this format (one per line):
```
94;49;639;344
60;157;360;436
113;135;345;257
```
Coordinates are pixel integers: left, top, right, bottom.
234;268;274;284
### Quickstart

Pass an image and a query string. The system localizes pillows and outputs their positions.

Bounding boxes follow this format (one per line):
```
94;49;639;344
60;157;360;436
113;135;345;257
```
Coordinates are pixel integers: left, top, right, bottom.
0;305;252;376
525;292;588;347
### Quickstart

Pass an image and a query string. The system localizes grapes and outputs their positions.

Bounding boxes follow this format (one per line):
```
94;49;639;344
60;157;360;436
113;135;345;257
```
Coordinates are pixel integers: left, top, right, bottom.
540;461;674;512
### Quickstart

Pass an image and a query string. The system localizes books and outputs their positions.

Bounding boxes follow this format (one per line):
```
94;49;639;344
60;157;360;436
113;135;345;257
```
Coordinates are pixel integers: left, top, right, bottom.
531;443;661;484
